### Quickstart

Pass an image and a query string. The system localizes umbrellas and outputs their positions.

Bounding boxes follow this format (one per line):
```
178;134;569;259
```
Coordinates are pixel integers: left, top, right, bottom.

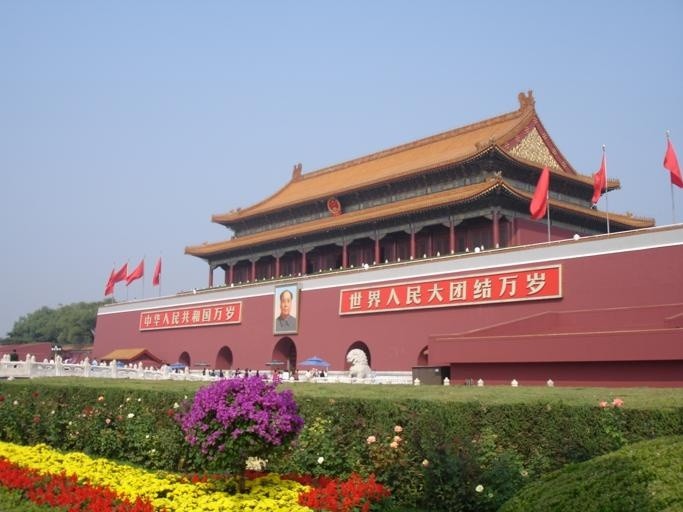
299;355;331;367
264;359;287;367
168;362;190;369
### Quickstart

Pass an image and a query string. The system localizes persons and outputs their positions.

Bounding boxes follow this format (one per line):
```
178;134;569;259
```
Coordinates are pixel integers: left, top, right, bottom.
65;356;71;364
276;289;297;331
9;348;19;368
203;367;325;382
79;357;125;368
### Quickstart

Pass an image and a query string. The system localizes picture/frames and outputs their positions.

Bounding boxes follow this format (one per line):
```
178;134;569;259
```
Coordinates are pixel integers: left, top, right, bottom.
273;282;301;336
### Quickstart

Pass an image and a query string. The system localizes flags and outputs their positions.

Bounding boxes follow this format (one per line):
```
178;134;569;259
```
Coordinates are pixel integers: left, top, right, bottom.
591;154;608;204
152;258;162;286
104;269;115;296
126;260;144;286
663;139;683;188
112;262;129;283
529;167;550;220
